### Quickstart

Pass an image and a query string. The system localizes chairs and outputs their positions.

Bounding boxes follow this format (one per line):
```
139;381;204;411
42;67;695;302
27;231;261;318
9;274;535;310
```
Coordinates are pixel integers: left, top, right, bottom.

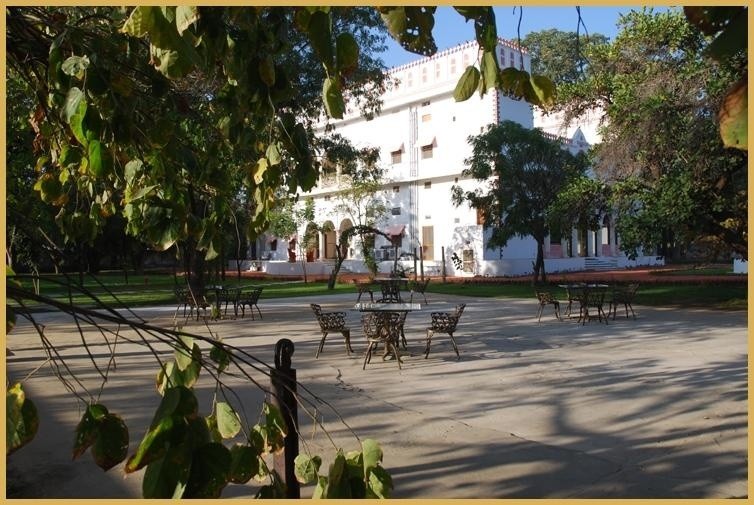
531;283;639;325
170;285;264;324
308;272;467;371
